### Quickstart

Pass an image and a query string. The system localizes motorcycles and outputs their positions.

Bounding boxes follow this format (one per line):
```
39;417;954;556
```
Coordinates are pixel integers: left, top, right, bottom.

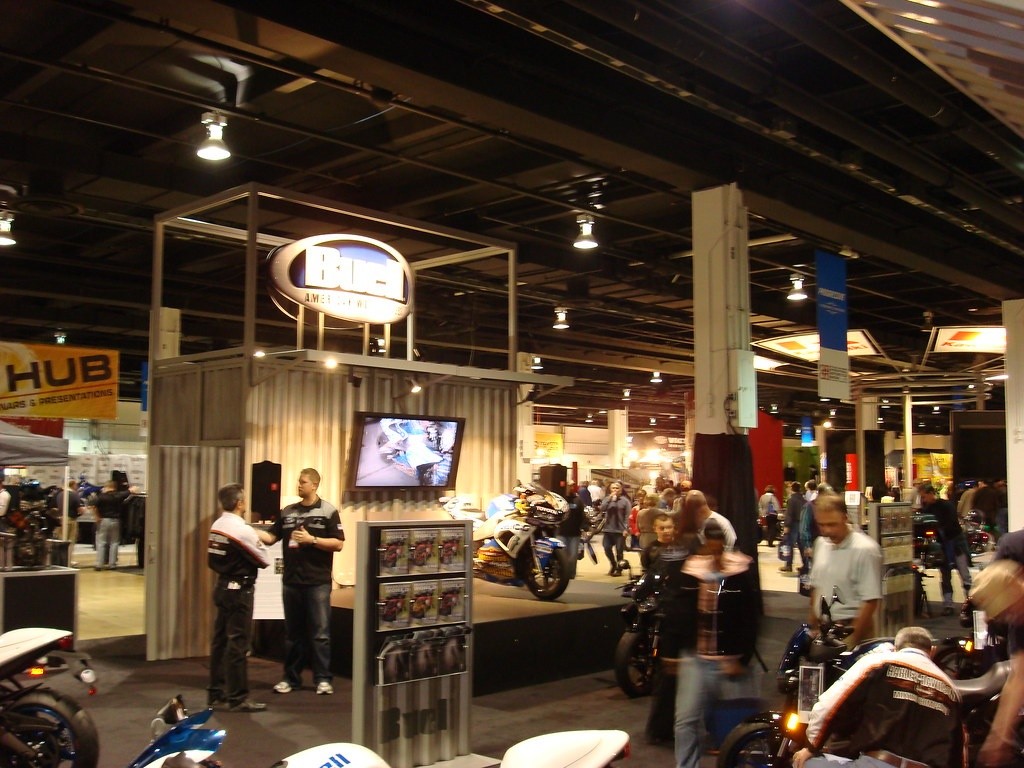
437;482;570;600
715;585;1024;768
912;510;988;569
0;626;100;768
615;559;756;697
128;694;629;768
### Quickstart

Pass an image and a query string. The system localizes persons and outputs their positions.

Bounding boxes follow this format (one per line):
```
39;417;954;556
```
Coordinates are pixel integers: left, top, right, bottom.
0;470;139;571
249;467;345;695
206;484;270;712
573;479;1024;768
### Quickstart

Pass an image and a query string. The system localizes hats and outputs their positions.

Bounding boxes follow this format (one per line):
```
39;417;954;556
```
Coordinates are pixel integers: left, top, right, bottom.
968;559;1024;622
511;479;522;489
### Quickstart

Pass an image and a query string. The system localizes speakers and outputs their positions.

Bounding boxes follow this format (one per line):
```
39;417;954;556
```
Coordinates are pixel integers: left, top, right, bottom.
252;460;281;514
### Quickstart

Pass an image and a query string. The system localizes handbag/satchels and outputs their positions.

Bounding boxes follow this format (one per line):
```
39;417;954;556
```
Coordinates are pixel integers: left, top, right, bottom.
766;495;778;516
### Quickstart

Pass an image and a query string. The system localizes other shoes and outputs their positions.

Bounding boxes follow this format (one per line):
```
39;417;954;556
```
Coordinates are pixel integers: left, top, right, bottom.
941;607;954;616
796;567;802;571
209;693;228;704
229;697;267;712
781;566;792;572
316;681;334;695
608;566;621;577
273;681;301;693
71;561;79;567
769;544;775;547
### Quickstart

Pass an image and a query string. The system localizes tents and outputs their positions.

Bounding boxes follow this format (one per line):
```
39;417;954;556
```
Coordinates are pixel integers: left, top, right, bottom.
0;419;69;541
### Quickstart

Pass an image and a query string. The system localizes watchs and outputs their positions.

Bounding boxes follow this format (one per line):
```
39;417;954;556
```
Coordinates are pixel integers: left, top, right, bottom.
312;536;317;547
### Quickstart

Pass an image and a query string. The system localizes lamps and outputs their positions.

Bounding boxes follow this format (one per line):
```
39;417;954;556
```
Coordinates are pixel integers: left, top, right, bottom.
786;276;809;302
574;208;598;252
407;378;421;393
583;372;1014;426
531;358;543;369
0;210;16;250
551;304;568;328
197;107;231;161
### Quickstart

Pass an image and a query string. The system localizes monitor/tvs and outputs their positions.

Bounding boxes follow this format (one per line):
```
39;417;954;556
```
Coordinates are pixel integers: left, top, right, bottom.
345;411;466;492
